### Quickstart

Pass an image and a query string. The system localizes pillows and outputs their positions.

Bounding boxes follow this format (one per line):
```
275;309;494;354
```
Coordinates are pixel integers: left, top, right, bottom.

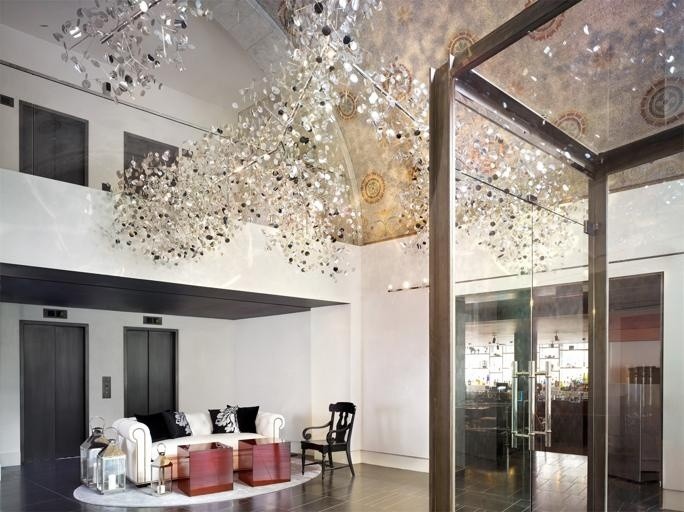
137;403;262;442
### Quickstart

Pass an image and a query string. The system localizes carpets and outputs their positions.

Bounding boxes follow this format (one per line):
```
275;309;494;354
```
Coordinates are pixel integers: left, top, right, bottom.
72;455;323;508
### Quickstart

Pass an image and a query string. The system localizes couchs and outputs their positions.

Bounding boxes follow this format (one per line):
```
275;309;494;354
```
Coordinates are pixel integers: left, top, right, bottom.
110;408;289;491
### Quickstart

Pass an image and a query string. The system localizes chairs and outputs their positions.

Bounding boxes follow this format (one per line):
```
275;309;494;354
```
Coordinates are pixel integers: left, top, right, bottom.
300;402;357;481
